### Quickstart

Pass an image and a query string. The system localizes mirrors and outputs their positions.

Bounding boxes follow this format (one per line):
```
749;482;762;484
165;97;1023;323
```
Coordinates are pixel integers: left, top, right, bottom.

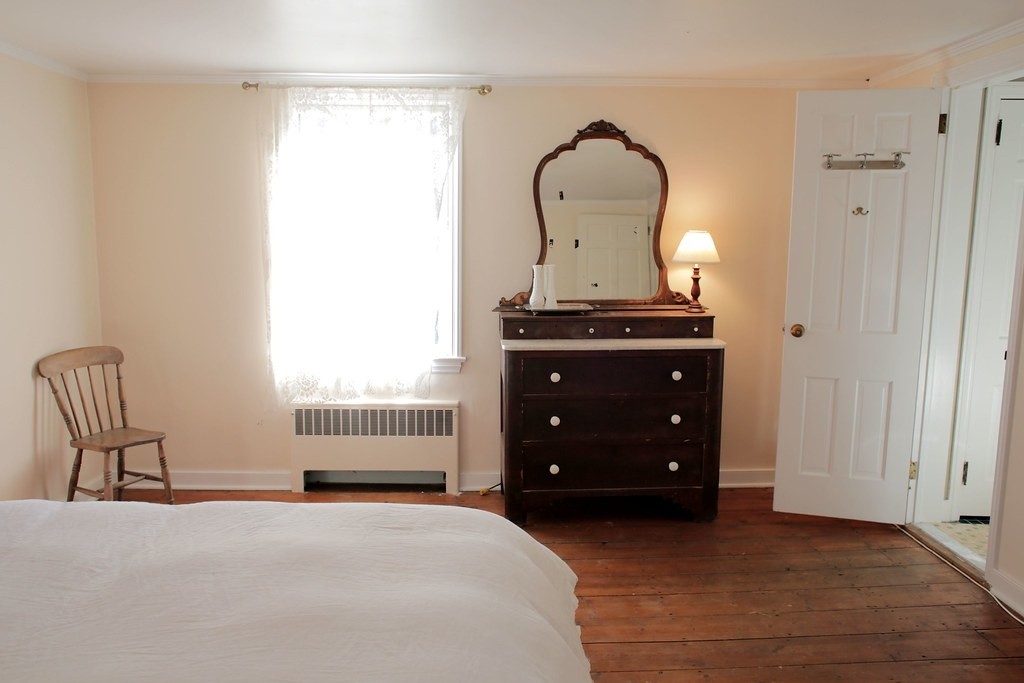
512;114;689;309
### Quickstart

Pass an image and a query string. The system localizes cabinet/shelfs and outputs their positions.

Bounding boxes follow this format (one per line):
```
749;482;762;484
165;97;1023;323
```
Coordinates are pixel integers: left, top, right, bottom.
499;307;718;340
498;338;728;525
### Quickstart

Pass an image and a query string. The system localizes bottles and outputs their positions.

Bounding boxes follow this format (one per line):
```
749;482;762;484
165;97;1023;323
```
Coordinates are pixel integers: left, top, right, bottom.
529;264;545;308
544;264;558;308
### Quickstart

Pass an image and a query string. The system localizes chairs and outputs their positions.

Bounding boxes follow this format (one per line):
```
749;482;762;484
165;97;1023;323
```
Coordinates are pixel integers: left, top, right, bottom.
36;345;175;508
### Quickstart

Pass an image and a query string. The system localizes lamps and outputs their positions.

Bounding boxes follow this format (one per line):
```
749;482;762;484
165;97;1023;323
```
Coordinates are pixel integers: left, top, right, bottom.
671;230;722;317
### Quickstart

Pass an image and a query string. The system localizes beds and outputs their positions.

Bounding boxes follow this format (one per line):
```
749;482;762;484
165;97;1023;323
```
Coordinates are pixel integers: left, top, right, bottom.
0;499;594;683
